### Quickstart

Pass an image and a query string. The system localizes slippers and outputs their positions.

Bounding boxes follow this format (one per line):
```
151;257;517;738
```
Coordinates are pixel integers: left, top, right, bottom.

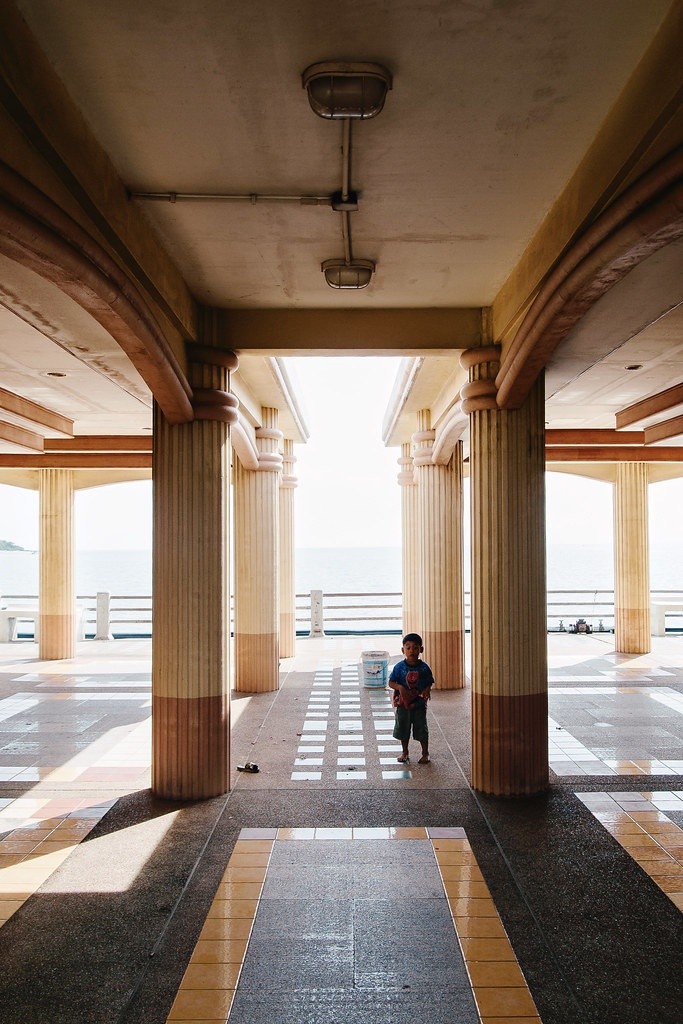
237;762;259;773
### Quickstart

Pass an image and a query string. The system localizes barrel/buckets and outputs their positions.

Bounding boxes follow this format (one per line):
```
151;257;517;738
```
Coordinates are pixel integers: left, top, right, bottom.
360;650;391;690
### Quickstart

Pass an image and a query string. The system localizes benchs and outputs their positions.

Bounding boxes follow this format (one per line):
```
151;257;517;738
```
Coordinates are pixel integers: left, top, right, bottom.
649;601;682;637
0;609;39;643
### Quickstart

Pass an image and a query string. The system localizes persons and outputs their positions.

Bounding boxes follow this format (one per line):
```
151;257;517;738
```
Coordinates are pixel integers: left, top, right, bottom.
389;633;435;764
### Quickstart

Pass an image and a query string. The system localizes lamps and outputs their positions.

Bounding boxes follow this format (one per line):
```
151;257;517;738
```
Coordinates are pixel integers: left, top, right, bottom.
300;59;392;120
321;259;374;289
